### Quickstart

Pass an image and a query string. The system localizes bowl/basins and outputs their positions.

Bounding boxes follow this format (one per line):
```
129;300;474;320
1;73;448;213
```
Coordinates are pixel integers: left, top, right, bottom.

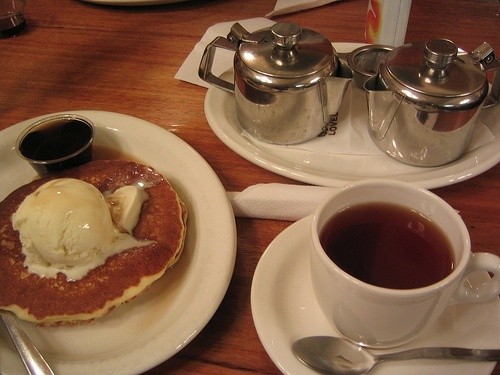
15;114;96;178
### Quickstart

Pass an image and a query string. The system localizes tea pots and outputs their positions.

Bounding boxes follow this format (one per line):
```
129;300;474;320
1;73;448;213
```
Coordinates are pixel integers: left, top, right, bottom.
198;22;354;145
363;38;500;167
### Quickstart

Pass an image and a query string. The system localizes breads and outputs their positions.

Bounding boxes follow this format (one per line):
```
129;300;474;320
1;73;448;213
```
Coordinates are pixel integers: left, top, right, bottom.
0;158;189;325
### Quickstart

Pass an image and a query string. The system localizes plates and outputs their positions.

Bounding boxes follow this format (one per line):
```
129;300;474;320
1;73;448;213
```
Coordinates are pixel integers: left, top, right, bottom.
204;42;500;190
251;214;499;374
0;110;237;375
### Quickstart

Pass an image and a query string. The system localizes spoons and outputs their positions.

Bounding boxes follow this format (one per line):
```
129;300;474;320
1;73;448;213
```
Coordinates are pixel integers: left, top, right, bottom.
292;336;500;374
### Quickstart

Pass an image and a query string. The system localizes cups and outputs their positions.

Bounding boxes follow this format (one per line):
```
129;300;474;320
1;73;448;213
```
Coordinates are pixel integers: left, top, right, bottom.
0;0;27;39
309;179;500;349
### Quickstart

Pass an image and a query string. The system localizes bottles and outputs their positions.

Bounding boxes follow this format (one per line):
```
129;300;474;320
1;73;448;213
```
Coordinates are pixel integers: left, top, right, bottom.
364;0;412;48
